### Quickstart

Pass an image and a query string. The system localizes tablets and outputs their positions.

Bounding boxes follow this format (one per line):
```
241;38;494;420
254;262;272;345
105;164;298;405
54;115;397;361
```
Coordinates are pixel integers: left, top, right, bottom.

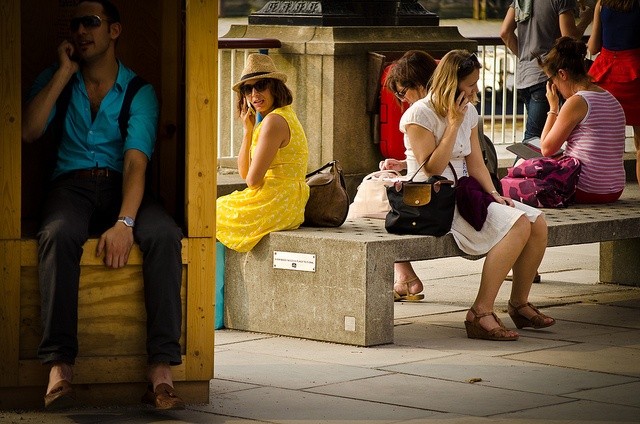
523;136;565;158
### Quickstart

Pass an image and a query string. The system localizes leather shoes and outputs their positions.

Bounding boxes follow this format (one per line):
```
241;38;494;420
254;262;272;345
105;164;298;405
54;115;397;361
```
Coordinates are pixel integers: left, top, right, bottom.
45;380;74;409
142;383;185;412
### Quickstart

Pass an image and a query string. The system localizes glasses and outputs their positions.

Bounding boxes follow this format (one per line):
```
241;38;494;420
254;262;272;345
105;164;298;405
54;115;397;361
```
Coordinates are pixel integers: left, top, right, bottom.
70;15;108;31
394;84;409;102
547;71;558;84
240;79;269;95
458;53;479;70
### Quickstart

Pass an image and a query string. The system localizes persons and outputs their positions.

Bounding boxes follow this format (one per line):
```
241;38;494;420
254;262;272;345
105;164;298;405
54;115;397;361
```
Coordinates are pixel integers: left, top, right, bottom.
379;51;445;300
399;50;556;341
216;53;310;252
500;0;593;166
540;37;627;204
22;1;185;413
586;0;640;190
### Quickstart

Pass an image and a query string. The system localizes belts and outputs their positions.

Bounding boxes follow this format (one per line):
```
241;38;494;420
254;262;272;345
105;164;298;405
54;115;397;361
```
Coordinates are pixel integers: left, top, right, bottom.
62;169;123;178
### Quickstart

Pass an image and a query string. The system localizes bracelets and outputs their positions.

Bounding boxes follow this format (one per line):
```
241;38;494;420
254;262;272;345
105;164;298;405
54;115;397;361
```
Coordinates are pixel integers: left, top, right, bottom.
489;190;500;195
546;111;559;117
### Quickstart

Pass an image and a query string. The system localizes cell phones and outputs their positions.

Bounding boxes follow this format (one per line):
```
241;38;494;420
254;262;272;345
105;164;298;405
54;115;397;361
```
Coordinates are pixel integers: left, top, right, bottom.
454;86;465;106
246;99;255;115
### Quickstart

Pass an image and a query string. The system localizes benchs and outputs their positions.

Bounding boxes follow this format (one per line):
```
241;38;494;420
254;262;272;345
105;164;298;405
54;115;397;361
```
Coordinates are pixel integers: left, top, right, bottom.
224;195;639;346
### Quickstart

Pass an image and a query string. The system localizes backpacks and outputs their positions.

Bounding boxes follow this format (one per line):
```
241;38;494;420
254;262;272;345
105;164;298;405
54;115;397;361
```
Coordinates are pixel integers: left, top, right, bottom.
500;157;581;208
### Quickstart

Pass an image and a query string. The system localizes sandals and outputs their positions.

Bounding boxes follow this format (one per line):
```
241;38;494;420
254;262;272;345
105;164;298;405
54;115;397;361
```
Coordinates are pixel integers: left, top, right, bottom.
465;308;519;340
504;270;540;283
508;300;556;329
393;277;424;301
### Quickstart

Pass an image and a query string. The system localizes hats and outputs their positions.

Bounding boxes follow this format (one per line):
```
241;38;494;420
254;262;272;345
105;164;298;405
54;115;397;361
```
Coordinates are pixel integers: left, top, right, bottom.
233;53;287;92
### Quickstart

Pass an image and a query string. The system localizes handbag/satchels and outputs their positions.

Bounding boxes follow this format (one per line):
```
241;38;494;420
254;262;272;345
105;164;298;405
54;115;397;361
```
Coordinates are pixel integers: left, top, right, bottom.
581;49;593;74
385;155;459;236
478;117;502;196
348;170;401;218
300;160;349;227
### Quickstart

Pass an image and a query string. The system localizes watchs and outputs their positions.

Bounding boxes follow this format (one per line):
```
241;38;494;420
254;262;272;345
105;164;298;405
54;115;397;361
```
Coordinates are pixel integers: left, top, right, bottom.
116;215;134;228
